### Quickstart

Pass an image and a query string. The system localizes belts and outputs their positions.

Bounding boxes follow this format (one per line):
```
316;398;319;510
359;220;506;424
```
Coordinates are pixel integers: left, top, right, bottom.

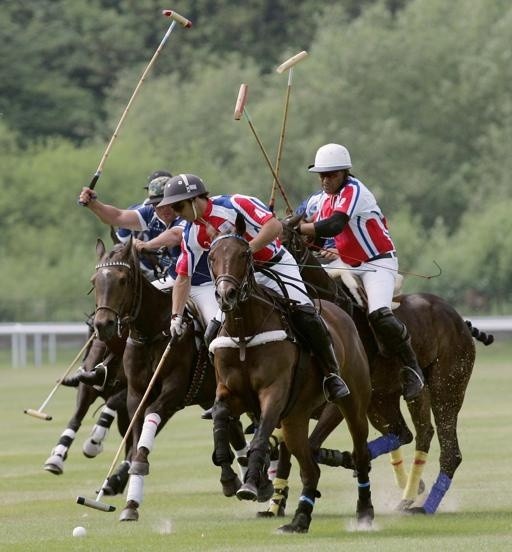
256;249;285;271
351;252;396;266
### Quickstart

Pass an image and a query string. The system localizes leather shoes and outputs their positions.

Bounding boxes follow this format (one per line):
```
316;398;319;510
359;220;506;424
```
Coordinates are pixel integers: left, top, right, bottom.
201;406;213;420
56;367;86;387
325;373;349;402
77;367;104;386
403;361;424;398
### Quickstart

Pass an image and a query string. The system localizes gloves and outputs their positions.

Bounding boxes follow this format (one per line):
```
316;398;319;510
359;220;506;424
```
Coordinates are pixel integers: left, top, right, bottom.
169;314;183;337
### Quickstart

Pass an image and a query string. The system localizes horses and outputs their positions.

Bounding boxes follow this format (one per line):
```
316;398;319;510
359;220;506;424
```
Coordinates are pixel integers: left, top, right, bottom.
92;225;274;523
198;211;376;534
277;212;495;517
43;225;144;495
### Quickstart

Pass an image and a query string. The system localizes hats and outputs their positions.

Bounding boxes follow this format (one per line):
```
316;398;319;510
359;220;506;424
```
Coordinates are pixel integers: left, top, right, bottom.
155;173;208;206
309;143;352;173
144;171;172;189
143;176;170;206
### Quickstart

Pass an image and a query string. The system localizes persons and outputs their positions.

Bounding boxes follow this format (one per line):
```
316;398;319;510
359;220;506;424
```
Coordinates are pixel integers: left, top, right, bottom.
78;176;225;421
58;364;86;389
293;143;426;401
157;172;351;436
